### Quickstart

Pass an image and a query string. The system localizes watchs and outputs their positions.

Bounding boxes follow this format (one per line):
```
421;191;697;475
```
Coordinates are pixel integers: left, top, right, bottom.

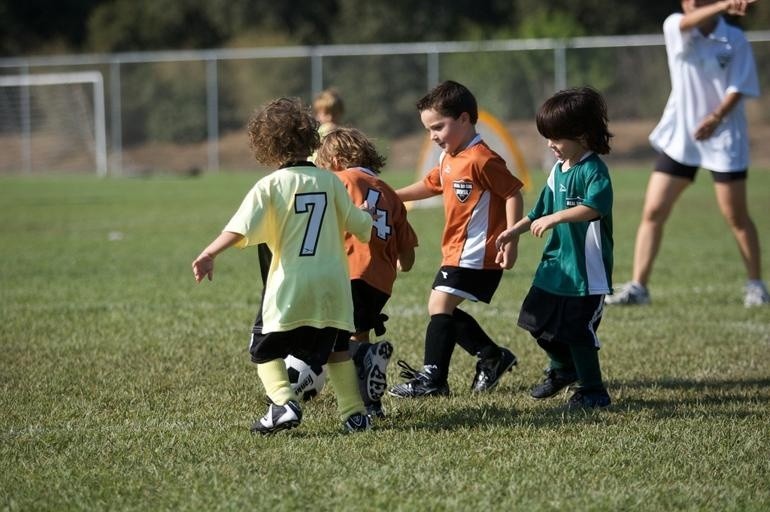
711;110;727;125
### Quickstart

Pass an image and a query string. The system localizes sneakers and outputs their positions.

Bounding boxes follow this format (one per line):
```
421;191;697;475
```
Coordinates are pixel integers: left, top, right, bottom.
568;390;612;410
388;359;451;398
743;282;769;309
250;397;303;431
364;339;393;401
342;411;372;434
470;346;517;396
604;281;652;305
528;368;576;398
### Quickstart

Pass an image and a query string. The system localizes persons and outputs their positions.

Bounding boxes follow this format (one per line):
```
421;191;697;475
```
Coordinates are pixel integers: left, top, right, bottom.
495;82;619;414
309;121;421;419
189;94;377;438
387;78;521;402
603;1;768;313
312;89;345;126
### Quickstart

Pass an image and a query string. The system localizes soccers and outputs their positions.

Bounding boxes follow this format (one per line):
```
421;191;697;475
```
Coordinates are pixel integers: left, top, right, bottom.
286;355;325;402
